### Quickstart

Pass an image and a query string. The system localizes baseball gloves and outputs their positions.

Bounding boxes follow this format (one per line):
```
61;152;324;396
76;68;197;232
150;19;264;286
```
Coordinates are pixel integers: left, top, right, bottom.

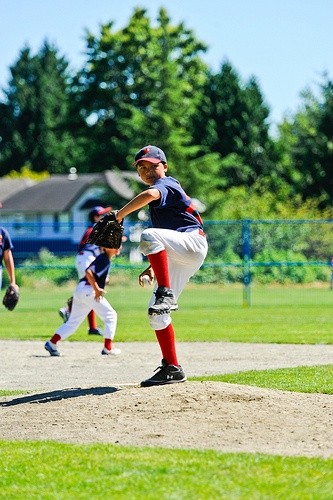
2;283;20;311
85;209;125;249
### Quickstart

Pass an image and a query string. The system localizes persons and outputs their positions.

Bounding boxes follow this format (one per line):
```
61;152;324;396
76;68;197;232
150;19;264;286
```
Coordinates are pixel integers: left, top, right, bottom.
59;206;112;335
85;144;209;387
44;242;122;355
0;202;20;311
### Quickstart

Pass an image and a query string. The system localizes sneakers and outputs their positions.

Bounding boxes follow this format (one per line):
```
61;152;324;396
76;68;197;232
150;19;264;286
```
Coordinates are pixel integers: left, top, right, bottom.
141;359;186;386
60;308;69;323
101;347;118;357
148;286;178;316
89;328;104;335
45;340;62;355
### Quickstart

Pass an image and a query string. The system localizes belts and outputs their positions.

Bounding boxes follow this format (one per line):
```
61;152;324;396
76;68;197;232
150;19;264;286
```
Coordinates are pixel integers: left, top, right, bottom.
198;229;205;236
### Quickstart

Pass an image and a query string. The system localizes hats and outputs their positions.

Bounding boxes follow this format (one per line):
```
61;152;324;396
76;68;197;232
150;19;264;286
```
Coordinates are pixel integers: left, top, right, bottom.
133;145;166;166
88;206;111;219
